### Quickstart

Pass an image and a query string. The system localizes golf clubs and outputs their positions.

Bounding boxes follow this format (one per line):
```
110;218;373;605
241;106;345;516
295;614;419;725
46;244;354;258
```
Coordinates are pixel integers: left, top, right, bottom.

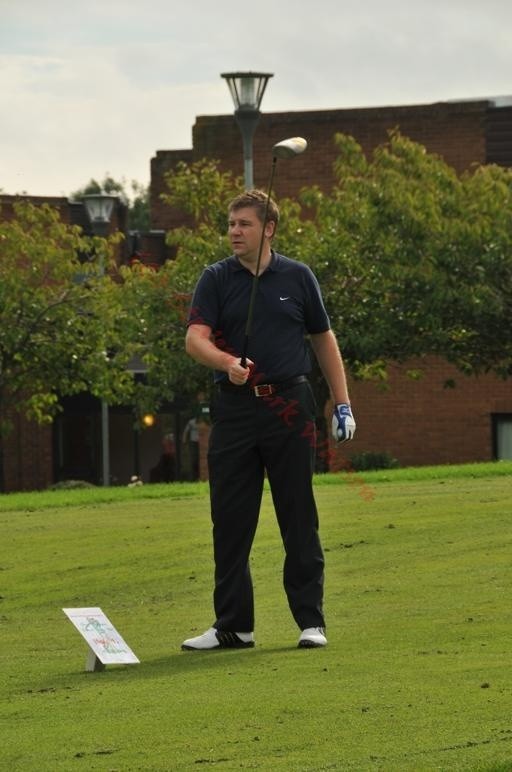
240;137;306;369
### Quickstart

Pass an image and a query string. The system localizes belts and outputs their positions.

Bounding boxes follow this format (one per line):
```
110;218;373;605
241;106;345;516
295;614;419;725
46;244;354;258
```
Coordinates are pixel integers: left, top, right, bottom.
221;374;310;397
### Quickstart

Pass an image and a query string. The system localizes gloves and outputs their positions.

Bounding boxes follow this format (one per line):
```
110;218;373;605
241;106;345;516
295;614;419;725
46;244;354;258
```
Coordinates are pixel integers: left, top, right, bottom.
331;402;356;445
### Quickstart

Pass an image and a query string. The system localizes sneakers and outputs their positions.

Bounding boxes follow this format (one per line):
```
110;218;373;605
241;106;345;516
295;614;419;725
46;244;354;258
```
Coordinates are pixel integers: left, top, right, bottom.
297;626;327;648
180;627;255;651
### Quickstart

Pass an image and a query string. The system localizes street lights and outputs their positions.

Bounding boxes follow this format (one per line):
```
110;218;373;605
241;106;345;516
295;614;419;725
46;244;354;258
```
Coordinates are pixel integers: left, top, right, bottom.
76;192;119;490
220;69;276;190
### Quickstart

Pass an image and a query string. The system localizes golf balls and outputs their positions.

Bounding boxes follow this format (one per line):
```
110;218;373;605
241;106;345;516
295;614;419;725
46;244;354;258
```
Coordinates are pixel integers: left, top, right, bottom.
338;429;343;437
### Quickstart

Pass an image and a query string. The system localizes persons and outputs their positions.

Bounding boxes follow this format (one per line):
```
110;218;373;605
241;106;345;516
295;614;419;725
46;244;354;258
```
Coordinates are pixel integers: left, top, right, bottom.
180;187;357;651
183;415;199;482
